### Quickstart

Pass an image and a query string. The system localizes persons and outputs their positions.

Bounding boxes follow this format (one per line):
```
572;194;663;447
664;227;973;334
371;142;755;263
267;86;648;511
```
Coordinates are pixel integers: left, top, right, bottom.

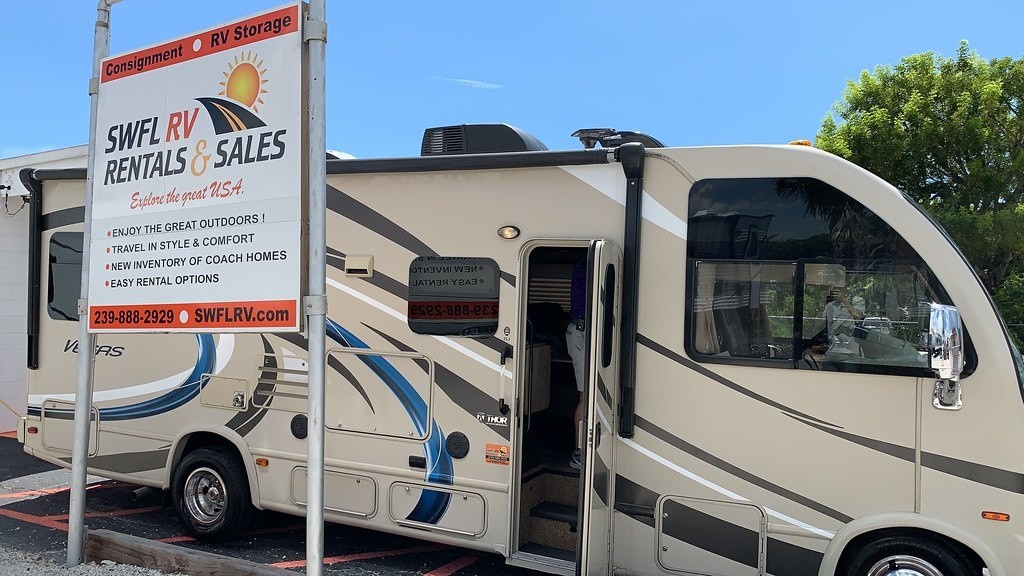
813;280;867;372
565;247;586;469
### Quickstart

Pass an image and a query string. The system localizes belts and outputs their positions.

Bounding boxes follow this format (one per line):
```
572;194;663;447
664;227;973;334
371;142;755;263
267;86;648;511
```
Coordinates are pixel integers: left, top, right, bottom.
570;318;578;325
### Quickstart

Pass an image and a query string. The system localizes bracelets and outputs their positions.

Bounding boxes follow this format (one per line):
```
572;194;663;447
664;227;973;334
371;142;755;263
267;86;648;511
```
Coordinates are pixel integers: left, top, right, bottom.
845;300;850;308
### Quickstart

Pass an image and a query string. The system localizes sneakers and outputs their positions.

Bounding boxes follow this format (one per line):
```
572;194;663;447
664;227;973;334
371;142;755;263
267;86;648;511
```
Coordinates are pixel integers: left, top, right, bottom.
569;450;581;470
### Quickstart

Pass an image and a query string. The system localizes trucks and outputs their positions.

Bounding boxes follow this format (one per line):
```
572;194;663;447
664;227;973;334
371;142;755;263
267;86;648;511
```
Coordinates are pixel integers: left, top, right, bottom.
17;122;1024;576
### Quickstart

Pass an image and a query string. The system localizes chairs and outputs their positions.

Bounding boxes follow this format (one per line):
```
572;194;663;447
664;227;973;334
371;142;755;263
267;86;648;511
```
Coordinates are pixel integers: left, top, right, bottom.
713;265;773;358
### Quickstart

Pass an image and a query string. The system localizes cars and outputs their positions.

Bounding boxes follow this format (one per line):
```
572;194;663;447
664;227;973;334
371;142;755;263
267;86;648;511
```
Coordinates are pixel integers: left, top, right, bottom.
862;317;892;334
